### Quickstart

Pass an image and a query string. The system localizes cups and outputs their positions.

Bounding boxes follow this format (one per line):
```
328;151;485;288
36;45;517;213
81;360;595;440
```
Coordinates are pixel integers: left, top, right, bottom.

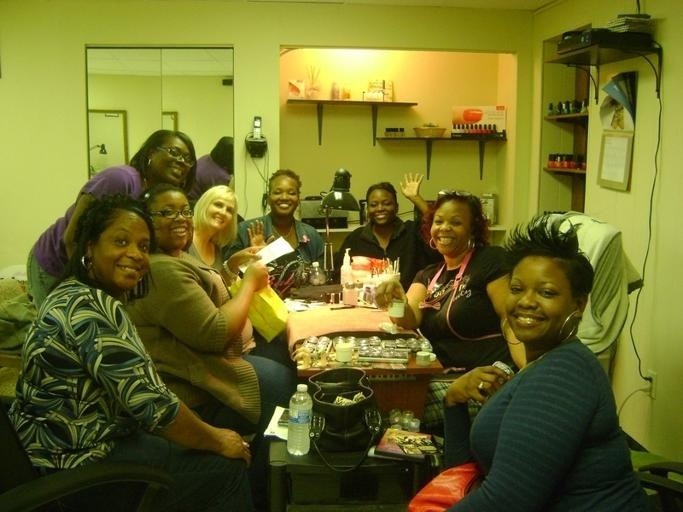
386;298;405;319
331;81;351;100
415;351;432;366
387;409;422;434
334;342;355;361
325;292;342;304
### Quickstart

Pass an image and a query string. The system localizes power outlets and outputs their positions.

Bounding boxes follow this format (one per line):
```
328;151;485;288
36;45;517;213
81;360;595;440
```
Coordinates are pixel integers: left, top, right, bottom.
641;368;658;400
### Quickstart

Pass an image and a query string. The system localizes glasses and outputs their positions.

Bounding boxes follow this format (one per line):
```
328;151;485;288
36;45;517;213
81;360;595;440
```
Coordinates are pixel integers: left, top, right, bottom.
434;190;477;209
152;209;192;219
158;148;195;168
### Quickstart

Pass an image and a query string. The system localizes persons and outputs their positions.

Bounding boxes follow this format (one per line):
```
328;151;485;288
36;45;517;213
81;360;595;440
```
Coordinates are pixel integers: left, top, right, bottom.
223;171;325;270
187;185;238;273
375;191;538;429
123;183;299;472
27;130;196;312
185;137;234;201
6;193;254;512
339;172;431;295
443;217;655;512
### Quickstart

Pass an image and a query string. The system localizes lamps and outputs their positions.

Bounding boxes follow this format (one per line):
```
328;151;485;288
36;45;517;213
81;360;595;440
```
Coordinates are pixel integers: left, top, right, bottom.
318;167;360;283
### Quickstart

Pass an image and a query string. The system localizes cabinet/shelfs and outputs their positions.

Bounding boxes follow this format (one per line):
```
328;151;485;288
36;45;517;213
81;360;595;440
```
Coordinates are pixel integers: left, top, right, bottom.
544;111;588;175
268;437;426;511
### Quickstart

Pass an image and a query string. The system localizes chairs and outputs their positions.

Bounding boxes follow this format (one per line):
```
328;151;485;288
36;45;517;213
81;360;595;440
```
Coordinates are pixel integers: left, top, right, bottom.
526;210;623;380
0;403;179;511
634;462;683;511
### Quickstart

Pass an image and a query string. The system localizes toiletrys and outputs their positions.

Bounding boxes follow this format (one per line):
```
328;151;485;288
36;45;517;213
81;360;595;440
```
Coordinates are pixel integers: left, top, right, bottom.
339;247;353;285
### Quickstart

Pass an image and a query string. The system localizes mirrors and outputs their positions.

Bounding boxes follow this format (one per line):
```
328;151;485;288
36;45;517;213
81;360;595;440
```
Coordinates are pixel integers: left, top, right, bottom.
84;46;235;199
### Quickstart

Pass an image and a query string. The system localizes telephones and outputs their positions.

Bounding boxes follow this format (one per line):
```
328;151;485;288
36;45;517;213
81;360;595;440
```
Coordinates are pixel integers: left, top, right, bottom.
246;115;267;158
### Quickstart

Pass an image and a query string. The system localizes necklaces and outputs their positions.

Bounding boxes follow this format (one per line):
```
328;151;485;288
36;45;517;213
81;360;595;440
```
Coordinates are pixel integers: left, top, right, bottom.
272;215;294;240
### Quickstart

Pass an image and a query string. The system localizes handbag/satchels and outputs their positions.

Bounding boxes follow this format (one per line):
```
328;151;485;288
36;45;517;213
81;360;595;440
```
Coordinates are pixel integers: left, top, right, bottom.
407;463;481;512
265;249;310;300
307;367;383;452
229;271;288;343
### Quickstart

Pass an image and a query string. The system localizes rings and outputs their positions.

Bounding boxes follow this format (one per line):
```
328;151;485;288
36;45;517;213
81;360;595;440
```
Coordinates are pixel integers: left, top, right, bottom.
242;441;250;448
478;381;483;389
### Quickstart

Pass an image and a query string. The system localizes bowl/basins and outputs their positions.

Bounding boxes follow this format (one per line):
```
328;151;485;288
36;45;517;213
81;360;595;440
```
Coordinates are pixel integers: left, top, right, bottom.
412;126;448;138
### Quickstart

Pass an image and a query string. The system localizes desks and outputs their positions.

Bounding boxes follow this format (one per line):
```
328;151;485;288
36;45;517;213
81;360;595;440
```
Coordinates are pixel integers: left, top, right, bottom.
315;224;508;233
285;293;444;425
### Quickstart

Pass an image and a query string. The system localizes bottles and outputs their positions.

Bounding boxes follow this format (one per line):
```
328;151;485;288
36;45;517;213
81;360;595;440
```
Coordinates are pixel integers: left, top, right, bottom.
546;98;587;115
308;260;327;285
342;281;357;305
362;285;373;304
545;152;584;171
285;383;314;459
355;282;364;301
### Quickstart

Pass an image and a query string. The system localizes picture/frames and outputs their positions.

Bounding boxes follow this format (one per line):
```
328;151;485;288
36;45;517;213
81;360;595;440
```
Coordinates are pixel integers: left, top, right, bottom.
162;111;178;131
596;129;634;191
88;109;129;180
